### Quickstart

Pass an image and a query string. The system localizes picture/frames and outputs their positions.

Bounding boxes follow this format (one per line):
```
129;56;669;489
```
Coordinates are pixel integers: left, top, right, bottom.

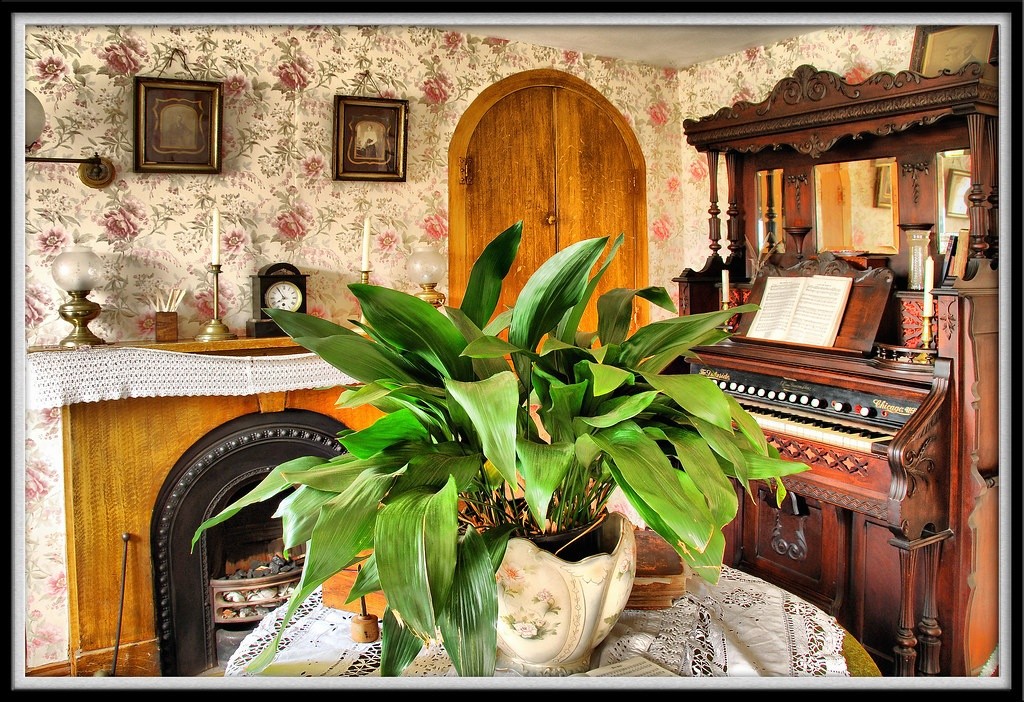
944;167;971;219
331;94;409;182
909;24;997;77
872;166;892;209
132;75;223;174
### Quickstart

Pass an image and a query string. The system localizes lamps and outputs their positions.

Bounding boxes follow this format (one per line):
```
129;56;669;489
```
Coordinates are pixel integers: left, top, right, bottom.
406;244;447;311
24;88;116;189
51;245;106;349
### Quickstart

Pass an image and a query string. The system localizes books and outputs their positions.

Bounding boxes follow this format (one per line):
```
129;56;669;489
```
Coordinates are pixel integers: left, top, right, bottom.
745;273;853;349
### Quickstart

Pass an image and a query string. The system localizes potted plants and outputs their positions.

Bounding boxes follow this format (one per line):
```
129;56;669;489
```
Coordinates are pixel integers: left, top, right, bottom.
188;221;812;676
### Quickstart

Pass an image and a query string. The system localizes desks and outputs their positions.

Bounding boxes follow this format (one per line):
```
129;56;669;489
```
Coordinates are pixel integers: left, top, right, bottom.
225;544;883;675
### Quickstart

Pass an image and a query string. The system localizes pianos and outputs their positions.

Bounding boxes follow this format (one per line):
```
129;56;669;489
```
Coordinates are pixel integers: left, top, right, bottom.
684;338;999;676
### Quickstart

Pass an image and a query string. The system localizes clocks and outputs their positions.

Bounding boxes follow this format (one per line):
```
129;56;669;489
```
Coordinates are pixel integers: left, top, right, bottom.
245;262;310;337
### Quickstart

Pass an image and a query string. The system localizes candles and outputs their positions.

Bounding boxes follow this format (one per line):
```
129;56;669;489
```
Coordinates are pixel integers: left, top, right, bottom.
722;269;730;301
361;215;371;271
924;255;935;317
211;206;221;265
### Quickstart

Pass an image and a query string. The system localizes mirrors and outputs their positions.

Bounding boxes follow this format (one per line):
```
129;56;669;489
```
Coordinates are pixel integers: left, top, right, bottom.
811;155;900;257
935;147;973;254
750;167;786;256
